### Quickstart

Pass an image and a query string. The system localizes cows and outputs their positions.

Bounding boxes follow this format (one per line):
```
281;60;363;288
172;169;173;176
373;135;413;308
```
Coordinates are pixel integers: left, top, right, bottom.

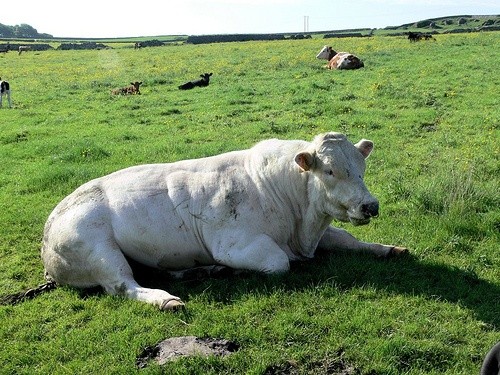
408;31;436;43
0;76;12;108
178;73;213;90
40;131;410;312
316;45;364;70
112;82;142;95
